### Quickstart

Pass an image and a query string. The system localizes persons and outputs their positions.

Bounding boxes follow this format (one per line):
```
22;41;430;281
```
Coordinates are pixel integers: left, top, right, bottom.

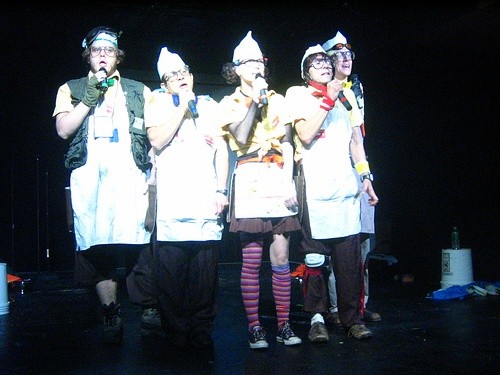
53;25;384;350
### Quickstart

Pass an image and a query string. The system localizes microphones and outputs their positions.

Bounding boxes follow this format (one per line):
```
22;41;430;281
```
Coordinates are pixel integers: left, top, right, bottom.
99;68;108;90
188;100;199;119
255;74;268;106
338;92;352;111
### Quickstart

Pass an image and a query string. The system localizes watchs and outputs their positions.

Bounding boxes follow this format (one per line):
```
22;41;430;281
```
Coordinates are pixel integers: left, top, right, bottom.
360;173;373;183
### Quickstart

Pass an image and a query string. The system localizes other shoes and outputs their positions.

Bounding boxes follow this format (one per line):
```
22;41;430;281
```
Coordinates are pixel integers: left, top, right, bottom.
327;312;343;326
138;308;169;340
308;322;329;344
364;308;382;321
348;324;374;339
103;303;128;345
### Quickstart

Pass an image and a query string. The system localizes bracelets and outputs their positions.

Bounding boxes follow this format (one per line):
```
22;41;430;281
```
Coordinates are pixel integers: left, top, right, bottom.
319;95;335;111
354;161;370;175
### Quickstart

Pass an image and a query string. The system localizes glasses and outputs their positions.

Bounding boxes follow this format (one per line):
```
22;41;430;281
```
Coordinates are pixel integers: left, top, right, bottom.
89;45;118;58
86;26;117;55
164;67;190;84
306;59;336;69
326;42;351;52
330;51;356;62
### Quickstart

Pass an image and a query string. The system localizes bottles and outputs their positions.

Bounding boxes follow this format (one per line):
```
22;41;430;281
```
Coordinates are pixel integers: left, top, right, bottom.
451;226;460;250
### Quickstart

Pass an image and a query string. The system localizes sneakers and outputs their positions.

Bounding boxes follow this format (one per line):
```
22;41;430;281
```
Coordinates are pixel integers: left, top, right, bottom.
276;325;302;346
247;325;269;349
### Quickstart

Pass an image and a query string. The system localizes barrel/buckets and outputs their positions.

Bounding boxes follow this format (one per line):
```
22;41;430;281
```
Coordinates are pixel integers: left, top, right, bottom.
440;247;474;289
0;263;10;315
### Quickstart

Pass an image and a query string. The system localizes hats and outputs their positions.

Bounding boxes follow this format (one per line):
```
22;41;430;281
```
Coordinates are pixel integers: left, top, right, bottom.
82;25;118;50
232;30;264;62
322;31;347;52
157;47;186;81
300;43;329;81
